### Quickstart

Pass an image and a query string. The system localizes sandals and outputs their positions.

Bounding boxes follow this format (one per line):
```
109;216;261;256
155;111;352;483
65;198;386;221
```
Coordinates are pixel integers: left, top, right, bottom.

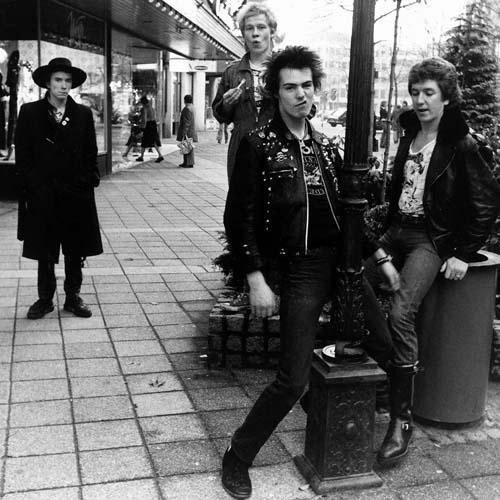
155;156;164;162
136;157;143;161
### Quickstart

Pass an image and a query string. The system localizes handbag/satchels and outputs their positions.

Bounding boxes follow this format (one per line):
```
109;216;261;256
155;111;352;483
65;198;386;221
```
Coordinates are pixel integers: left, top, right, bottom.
177;134;195;155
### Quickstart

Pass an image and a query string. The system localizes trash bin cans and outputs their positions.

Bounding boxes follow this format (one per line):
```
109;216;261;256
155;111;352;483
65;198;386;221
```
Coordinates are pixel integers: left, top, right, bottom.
411;246;500;429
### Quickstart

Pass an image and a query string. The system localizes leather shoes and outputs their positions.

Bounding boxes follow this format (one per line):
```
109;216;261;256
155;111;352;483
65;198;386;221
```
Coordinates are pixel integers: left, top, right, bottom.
221;447;252;499
27;299;54;319
64;297;92;318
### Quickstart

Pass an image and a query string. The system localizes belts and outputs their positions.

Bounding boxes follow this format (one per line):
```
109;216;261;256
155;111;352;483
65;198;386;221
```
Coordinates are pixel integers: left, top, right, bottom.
308;247;336;257
396;211;426;223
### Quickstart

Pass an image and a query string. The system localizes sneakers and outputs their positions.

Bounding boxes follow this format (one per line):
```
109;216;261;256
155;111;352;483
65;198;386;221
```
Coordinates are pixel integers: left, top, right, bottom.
220;292;250;313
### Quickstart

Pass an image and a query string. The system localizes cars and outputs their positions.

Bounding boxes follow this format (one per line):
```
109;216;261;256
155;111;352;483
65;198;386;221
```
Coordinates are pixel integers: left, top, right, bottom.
317;107;382;130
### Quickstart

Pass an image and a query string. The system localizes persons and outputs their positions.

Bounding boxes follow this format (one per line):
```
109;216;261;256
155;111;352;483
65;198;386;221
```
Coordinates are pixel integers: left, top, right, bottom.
391;104;402;143
361;55;499;462
396;100;410;137
214;122;229;144
379;101;393;148
212;0;280;312
219;43;401;500
15;56;102;320
121;94;164;164
176;94;199;168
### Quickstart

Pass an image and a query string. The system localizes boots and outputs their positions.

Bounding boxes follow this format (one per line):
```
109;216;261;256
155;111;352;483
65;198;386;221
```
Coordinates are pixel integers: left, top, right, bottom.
376;361;420;463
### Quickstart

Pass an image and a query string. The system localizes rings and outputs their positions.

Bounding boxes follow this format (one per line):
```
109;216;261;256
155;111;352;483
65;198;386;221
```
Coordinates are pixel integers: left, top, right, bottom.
455;274;461;279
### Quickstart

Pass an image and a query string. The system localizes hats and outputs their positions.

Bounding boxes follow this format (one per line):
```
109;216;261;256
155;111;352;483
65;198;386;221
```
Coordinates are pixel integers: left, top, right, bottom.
32;57;87;89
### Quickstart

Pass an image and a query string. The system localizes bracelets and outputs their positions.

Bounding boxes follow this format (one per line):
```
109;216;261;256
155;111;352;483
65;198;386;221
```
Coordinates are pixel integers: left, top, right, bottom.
374;254;394;267
